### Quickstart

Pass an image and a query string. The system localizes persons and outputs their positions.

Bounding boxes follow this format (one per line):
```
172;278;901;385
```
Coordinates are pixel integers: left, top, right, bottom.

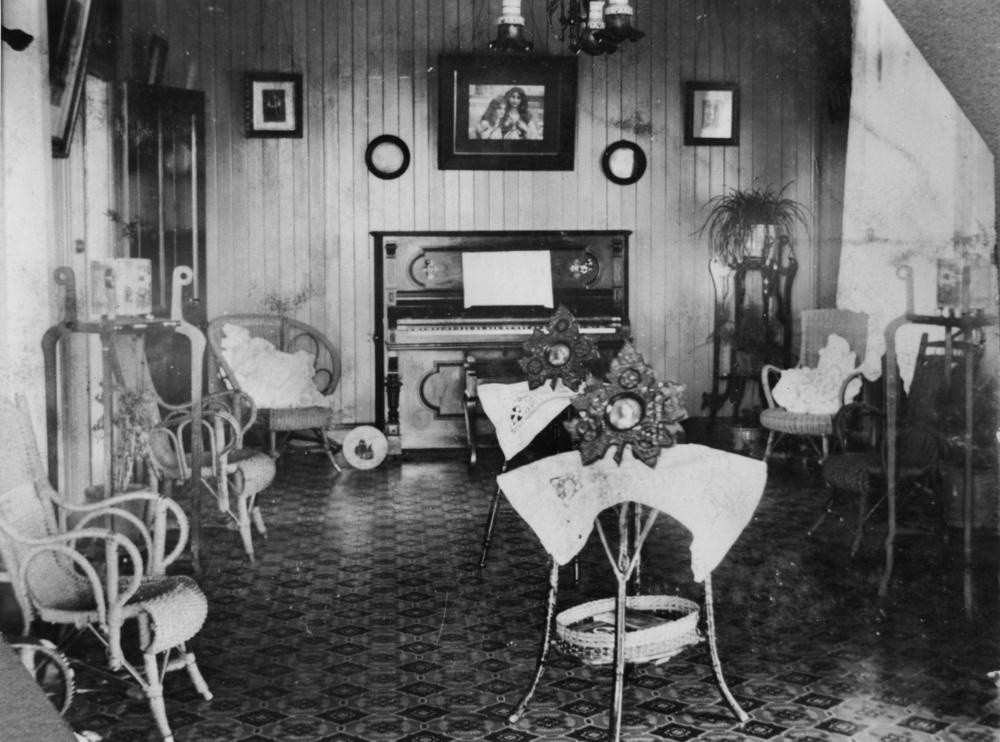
475;87;539;140
266;93;283;120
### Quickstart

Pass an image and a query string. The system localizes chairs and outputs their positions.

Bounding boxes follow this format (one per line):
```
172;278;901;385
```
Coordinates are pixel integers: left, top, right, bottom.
761;305;874;473
0;630;102;742
801;332;989;557
206;312;345;473
102;317;277;570
0;392;215;742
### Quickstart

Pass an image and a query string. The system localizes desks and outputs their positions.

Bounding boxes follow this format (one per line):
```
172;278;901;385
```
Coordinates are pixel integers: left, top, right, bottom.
496;440;769;742
476;377;615;569
699;252;801;425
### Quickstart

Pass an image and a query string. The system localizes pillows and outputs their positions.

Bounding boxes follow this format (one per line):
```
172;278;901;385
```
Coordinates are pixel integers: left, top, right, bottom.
218;324;332;410
771;333;864;417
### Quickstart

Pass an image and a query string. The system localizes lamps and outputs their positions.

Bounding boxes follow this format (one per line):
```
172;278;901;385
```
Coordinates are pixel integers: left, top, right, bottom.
485;0;649;57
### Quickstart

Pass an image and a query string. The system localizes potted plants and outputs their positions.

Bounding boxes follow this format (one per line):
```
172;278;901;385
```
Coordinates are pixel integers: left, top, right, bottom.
687;180;818;270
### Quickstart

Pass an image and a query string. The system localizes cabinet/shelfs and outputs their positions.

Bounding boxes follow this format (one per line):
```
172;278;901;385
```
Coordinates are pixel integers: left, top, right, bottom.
112;80;206;320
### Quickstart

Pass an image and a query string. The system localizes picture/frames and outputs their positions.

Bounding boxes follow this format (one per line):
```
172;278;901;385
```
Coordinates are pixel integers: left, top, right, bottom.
243;69;306;140
437;52;580;172
683;77;741;149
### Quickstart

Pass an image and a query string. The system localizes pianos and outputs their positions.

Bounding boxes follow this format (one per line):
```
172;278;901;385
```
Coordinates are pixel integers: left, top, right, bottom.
368;227;633;465
